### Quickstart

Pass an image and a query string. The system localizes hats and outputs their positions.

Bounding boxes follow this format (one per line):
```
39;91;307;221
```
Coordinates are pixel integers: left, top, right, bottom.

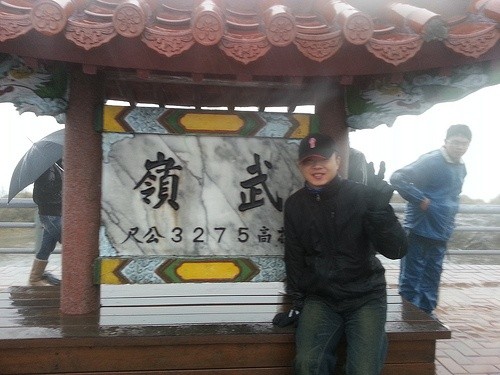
298;134;336;162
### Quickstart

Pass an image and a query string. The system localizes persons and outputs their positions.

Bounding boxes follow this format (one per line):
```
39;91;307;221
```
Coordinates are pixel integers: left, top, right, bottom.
30;158;62;287
391;124;473;328
273;133;409;375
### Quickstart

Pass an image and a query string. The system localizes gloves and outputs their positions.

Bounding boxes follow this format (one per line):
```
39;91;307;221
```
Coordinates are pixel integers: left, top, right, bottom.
274;299;303;328
367;162;393;202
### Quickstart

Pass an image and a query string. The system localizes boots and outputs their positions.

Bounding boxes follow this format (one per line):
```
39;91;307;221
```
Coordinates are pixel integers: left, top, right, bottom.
28;258;51;286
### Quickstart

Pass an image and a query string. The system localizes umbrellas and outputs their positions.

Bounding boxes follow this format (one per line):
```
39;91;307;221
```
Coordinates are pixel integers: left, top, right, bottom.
7;129;65;203
